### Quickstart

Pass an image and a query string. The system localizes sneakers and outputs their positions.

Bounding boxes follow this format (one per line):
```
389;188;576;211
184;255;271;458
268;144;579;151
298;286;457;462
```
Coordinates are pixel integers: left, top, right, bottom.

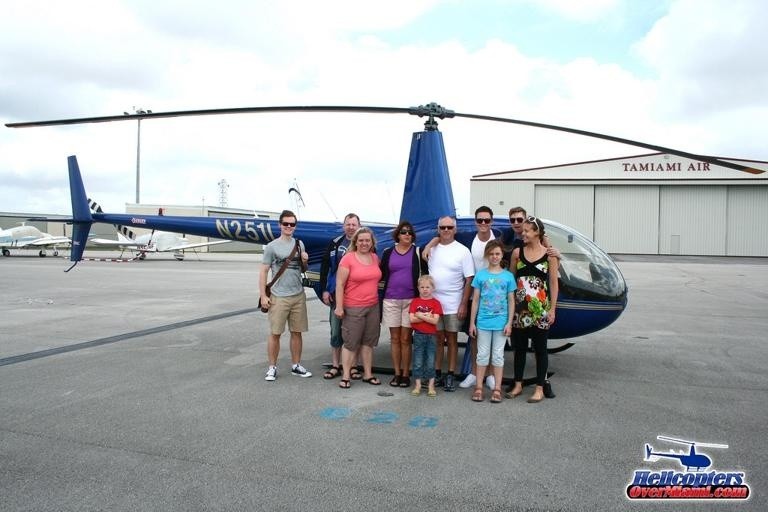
289;365;313;378
263;365;277;382
541;379;556;399
459;374;477;388
484;374;495;390
420;375;444;389
442;373;457;393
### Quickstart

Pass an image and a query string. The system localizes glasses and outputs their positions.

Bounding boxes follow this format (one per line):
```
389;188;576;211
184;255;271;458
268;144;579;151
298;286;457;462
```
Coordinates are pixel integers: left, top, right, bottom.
527;216;539;230
509;218;524;223
475;218;490;224
399;229;413;235
437;225;454;231
280;222;297;227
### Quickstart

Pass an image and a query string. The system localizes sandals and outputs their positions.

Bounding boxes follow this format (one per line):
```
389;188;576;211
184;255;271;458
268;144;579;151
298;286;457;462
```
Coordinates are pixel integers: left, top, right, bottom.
425;389;439;397
527;392;544;404
488;388;501;404
504;387;521;400
410;386;423;397
348;367;361;380
400;376;411;388
323;365;340;380
470;386;484;403
388;375;400;388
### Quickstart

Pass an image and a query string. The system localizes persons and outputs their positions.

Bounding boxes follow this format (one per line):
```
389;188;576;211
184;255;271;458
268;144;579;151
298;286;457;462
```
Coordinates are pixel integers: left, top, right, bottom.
422;216;475;392
469;240;517;403
459;206;502;391
409;276;444;396
318;213;377;380
259;211;313;381
334;227;382;388
379;221;440;387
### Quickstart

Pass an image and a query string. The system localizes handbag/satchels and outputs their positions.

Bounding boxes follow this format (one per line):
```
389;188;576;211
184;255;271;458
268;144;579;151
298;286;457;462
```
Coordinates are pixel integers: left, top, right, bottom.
257;278;278;313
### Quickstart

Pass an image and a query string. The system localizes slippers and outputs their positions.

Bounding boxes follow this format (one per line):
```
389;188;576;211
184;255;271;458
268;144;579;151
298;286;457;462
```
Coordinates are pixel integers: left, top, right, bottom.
338;377;351;389
361;376;382;386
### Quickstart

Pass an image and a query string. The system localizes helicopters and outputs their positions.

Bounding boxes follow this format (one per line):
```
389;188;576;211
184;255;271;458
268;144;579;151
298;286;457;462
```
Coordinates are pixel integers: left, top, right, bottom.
0;223;72;258
4;102;764;385
84;197;235;260
